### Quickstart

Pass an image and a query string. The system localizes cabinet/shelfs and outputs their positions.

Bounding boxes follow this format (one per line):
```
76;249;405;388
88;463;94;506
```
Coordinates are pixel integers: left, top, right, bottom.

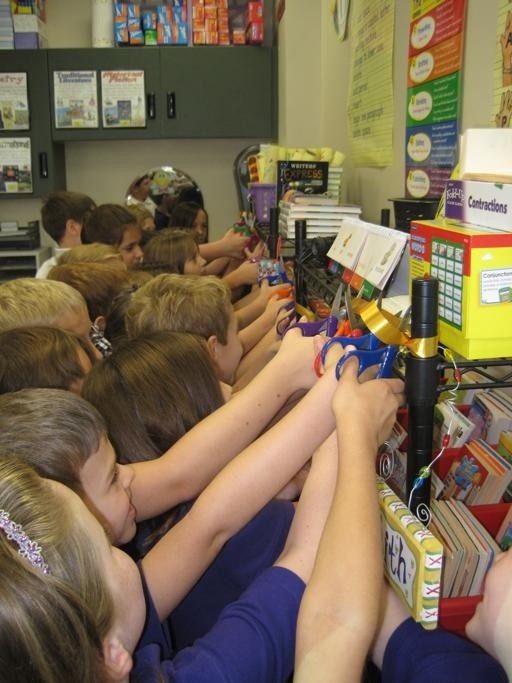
0;44;277;199
1;245;53;282
246;194;512;639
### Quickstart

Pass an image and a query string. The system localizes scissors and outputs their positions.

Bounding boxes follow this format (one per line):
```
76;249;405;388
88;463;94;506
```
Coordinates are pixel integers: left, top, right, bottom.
233;213;411;380
